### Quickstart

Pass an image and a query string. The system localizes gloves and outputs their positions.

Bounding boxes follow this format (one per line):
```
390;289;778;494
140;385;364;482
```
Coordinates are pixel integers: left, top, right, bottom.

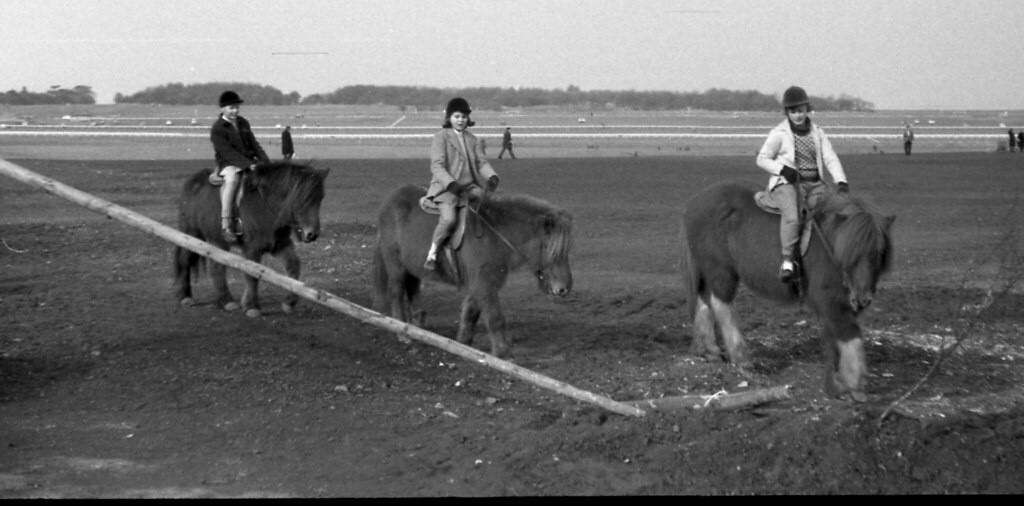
837;182;848;194
447;182;467;198
489;176;498;189
780;165;803;184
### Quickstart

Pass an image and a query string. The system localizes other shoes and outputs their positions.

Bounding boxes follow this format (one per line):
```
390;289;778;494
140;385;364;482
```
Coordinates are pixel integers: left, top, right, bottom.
781;260;794;281
220;225;238;243
424;254;438;271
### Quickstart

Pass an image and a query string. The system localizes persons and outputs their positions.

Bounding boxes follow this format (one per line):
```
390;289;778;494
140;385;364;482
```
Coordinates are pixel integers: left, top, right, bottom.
498;127;515;160
1008;129;1024;152
282;126;294;160
757;87;849;283
209;90;268;242
423;98;500;270
903;125;914;155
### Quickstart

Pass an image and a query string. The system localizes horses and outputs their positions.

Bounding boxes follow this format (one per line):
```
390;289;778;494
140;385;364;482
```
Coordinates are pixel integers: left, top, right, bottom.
367;185;578;366
677;177;897;401
173;160;332;317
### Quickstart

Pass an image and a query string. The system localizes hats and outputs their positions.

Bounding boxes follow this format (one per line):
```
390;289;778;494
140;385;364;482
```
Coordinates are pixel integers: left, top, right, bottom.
783;87;809;108
220;91;243;107
447;98;471;114
